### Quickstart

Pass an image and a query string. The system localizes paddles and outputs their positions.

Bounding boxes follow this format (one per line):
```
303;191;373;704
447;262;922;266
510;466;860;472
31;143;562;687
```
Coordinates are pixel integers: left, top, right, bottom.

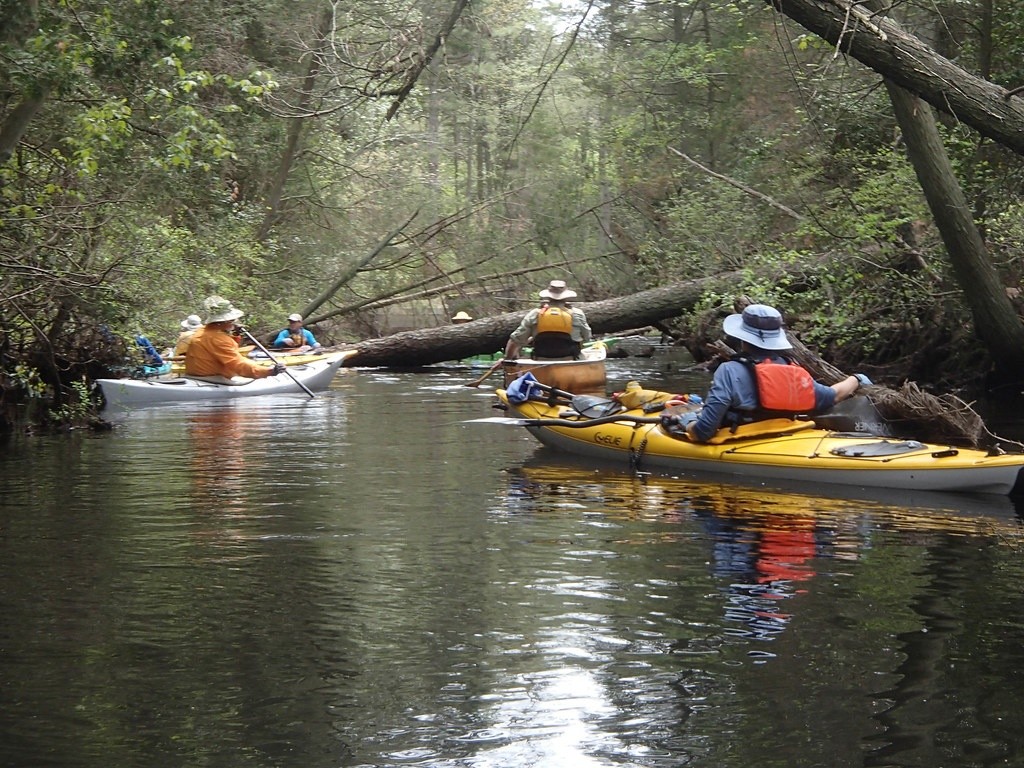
460;412;675;429
238;326;318;398
464;356;508;389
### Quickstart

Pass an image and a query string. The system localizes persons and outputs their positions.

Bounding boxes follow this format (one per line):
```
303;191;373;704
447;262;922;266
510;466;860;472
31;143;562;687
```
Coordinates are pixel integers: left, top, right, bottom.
184;295;287;379
272;313;321;350
173;314;204;356
502;280;593;361
710;525;858;644
675;303;873;442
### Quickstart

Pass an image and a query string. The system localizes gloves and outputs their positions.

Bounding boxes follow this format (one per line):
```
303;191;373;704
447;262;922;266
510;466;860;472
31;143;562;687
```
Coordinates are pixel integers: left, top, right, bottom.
676;412;698;426
234;324;248;333
851;374;873;387
269;363;286;374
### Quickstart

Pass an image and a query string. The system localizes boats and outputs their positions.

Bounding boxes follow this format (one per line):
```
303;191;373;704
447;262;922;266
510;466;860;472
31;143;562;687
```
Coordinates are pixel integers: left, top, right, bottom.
95;349;346;406
500;342;608;391
492;379;1024;496
171;349;359;380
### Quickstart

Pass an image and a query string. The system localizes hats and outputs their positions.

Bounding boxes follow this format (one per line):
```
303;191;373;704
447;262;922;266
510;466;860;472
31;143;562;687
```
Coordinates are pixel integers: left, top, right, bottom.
205;300;244;323
451;311;473;319
539;280;578;300
723;304;793;350
286;314;302;322
181;315;203;329
